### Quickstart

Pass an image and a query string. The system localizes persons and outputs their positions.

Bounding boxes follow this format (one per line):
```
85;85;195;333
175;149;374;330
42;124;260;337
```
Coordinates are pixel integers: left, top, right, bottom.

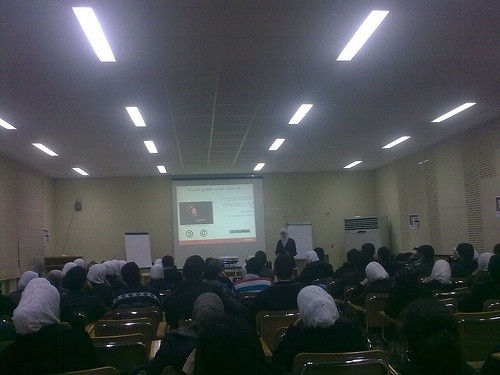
188;206;206;224
398;298;479;375
152;292;225;369
0;277;100;375
480;344;500;375
278;284;368;360
0;243;500;326
161;314;288;375
275;227;297;256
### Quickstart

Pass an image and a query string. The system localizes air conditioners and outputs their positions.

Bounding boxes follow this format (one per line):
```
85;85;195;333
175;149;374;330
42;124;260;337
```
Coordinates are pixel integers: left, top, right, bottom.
343;216;391;263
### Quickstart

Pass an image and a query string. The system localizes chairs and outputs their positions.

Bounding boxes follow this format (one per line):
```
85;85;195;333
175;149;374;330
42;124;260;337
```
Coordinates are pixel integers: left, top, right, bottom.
66;273;500;375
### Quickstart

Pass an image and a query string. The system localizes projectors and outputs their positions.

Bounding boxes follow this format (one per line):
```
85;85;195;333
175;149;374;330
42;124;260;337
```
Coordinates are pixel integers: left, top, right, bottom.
219;256;238;263
